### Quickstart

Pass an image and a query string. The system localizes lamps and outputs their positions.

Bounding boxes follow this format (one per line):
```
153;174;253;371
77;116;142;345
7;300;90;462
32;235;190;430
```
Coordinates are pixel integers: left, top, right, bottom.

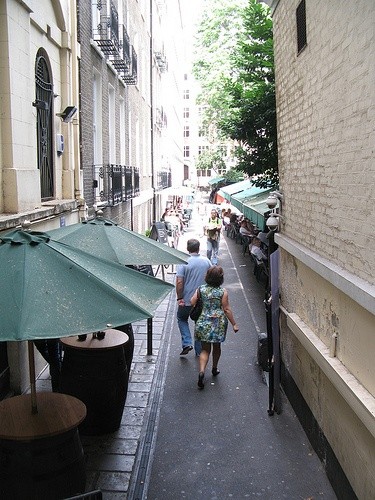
56;106;77;123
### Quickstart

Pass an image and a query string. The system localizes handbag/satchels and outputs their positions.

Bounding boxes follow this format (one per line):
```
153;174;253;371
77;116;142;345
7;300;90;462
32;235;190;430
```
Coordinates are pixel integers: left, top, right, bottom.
189;286;202;321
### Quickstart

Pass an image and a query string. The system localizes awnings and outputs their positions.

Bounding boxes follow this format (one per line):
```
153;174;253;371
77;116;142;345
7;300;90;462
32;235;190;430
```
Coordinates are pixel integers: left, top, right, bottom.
208;176;280;230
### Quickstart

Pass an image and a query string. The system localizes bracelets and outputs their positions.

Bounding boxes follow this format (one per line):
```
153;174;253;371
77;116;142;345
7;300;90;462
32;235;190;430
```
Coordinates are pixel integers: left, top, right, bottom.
177;297;183;301
233;323;236;327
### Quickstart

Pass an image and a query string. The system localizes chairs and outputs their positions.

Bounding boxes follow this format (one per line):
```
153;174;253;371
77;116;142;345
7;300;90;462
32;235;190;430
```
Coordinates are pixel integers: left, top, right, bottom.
225;225;269;289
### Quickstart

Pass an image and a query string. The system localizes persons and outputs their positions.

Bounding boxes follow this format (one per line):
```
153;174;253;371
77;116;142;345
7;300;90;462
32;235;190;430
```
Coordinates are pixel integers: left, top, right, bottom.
174;238;214;357
161;197;269;265
190;265;239;388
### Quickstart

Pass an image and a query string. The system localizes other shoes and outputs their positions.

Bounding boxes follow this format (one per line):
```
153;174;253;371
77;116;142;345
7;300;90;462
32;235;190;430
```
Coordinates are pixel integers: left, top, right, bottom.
180;345;193;355
212;369;220;376
197;372;205;389
196;350;203;356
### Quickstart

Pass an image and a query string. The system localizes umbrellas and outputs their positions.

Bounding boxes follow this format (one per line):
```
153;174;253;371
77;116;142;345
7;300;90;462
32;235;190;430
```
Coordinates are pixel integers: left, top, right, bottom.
0;210;192;416
